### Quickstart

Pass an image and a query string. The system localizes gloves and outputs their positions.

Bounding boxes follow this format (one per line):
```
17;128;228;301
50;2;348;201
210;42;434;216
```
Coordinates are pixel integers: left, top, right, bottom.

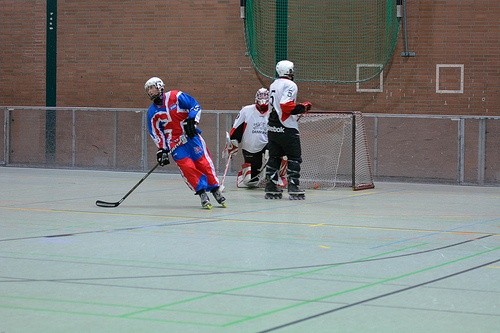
156;148;170;167
301;99;312;112
226;139;238;157
183;119;198;140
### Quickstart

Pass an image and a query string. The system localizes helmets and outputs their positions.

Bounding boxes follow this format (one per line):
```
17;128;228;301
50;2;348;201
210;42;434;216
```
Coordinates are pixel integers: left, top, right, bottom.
144;77;165;100
255;87;269;106
276;60;295;80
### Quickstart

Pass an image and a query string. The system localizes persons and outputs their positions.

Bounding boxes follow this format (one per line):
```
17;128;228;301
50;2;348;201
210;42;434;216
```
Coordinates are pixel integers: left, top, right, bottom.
228;88;288;189
264;60;313;196
144;77;227;208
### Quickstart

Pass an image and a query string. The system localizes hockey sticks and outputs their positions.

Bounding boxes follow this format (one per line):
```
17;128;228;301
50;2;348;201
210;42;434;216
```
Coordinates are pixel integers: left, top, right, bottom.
294;113;302;124
219;154;231;194
95;142;181;208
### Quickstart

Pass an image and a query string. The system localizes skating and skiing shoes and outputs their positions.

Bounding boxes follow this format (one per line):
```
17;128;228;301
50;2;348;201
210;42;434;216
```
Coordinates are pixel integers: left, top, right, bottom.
200;193;212;209
264;181;284;200
213;191;228;208
288;184;306;200
